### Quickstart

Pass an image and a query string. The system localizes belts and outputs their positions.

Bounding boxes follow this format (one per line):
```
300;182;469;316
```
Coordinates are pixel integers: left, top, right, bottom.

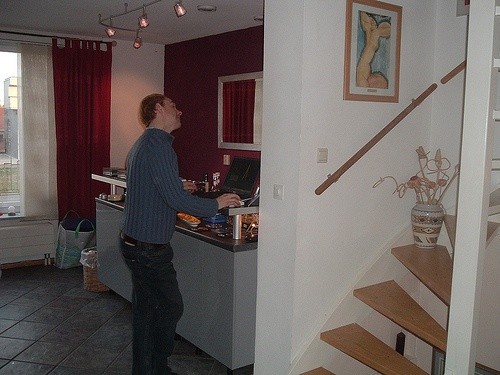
120;230;163;249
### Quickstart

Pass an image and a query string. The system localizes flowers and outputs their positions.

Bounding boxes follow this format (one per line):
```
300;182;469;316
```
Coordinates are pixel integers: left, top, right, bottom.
373;146;460;205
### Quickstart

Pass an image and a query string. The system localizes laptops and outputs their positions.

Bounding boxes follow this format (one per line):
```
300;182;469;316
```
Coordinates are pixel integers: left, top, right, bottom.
195;157;260;199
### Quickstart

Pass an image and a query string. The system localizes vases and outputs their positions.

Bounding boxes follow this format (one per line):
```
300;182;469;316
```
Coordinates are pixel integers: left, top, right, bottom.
410;201;445;249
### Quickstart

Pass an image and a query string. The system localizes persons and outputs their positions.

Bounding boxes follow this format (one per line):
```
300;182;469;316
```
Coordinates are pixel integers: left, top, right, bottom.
119;93;241;375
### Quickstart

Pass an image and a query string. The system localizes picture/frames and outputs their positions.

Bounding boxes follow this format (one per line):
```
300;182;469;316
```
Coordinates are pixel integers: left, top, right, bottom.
343;0;404;104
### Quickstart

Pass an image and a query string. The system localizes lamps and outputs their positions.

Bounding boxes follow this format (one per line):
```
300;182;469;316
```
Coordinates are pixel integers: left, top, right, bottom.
134;32;143;48
104;19;116;38
173;0;187;18
138;5;150;28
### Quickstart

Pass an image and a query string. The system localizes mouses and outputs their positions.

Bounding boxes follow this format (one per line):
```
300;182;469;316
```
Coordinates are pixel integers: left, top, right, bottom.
229;200;244;208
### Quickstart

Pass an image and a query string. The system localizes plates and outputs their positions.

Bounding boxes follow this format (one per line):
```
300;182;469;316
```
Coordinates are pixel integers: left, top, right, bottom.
176;213;201;227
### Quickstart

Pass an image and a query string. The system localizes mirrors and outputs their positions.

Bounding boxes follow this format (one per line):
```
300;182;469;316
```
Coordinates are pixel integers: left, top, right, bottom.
218;71;264;152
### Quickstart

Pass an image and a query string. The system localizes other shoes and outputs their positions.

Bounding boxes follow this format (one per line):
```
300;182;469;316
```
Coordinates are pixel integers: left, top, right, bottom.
152;366;189;375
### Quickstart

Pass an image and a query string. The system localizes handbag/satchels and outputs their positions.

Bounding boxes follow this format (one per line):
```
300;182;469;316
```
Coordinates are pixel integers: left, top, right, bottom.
53;210;96;270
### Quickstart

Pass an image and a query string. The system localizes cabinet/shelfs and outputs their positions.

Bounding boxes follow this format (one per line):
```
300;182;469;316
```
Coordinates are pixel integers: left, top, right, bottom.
91;173;261;375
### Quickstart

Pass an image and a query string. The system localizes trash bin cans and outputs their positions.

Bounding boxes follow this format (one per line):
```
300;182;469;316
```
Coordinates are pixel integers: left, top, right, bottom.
79;246;111;293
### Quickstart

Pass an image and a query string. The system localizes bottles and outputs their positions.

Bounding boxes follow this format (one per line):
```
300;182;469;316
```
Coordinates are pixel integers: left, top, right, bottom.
203;171;220;193
